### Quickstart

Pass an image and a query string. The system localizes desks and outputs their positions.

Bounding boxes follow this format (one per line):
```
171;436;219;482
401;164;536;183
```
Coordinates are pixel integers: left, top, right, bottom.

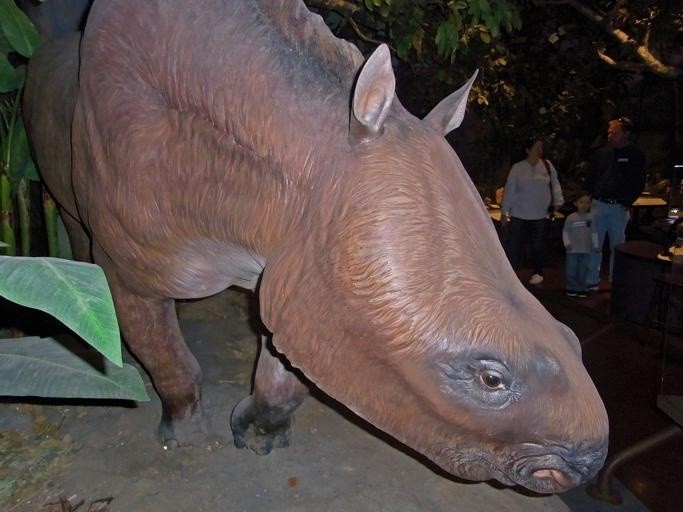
614;239;683;333
632;171;670;222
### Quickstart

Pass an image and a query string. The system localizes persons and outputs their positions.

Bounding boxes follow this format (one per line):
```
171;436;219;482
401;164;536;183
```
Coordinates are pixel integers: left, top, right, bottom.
586;116;647;294
561;190;601;299
500;137;566;285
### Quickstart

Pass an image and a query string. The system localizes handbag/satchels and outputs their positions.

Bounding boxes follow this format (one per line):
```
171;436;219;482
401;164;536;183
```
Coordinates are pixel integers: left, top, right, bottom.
542;211;563;250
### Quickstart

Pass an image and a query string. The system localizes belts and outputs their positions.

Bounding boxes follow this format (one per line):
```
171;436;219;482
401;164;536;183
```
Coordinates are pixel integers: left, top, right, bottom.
593;196;621;205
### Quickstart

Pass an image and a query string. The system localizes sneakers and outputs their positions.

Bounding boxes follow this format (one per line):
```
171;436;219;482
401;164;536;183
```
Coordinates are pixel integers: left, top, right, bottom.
528;274;544;285
584;283;602;291
564;287;588;299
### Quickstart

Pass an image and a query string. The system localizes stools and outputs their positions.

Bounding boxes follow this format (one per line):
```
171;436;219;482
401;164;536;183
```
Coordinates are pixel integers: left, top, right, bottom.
642;274;683;395
